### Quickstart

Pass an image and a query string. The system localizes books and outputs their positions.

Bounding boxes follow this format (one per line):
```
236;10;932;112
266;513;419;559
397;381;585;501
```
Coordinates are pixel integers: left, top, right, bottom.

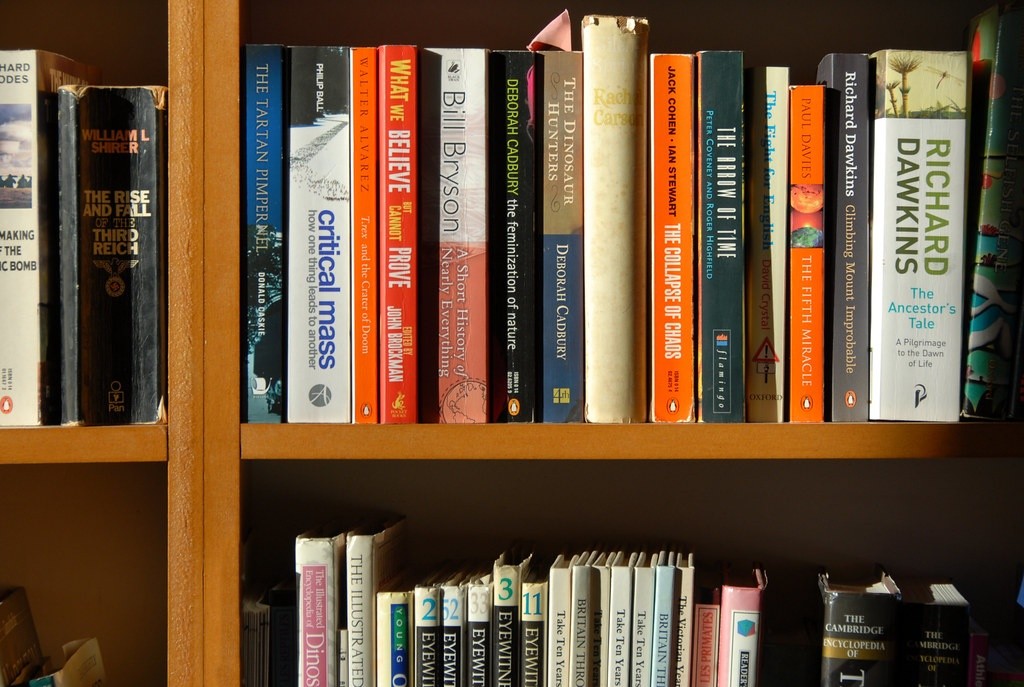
0;586;104;687
241;15;1024;426
0;49;168;429
241;522;1024;687
